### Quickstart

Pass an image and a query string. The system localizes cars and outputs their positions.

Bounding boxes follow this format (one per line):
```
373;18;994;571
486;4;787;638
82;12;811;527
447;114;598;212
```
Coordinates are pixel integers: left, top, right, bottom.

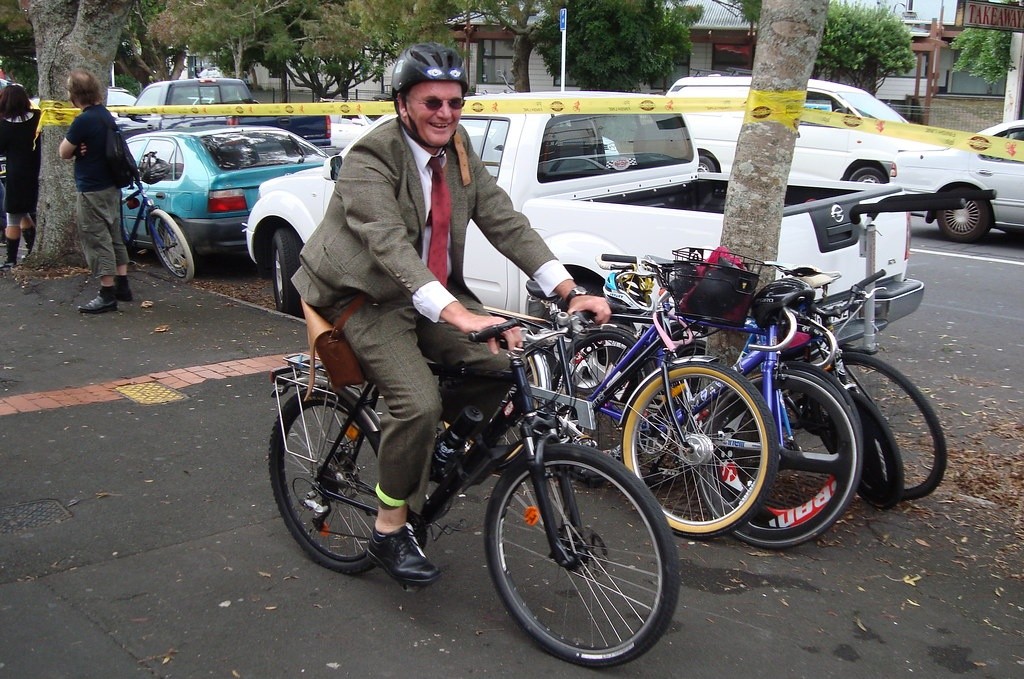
122;126;332;270
317;97;389;149
106;86;138;118
890;118;1024;243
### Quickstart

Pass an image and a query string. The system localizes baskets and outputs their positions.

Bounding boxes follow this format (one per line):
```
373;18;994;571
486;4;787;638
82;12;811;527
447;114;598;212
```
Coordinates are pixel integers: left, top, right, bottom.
672;246;765;330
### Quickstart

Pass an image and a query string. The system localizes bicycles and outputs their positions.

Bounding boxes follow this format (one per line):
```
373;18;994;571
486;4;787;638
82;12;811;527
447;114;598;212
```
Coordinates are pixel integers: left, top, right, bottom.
117;151;195;283
266;302;680;666
484;246;947;550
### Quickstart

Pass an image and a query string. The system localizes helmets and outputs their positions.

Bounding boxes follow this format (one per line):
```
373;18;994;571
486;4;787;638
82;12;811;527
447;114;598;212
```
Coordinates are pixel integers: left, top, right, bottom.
141;158;171;185
603;270;654;316
391;41;468;96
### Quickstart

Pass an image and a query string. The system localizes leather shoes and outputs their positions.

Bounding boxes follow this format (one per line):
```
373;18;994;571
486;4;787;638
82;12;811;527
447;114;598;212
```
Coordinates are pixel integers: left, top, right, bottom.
78;295;117;315
116;286;133;301
366;525;442;586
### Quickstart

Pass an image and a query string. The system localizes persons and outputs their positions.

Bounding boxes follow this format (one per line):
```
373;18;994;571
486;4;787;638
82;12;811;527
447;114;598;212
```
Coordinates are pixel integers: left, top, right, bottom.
291;43;616;583
0;79;41;272
58;70;133;315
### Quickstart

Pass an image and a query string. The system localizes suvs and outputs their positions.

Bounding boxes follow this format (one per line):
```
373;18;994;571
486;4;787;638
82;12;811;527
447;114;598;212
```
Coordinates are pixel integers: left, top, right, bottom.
667;73;909;184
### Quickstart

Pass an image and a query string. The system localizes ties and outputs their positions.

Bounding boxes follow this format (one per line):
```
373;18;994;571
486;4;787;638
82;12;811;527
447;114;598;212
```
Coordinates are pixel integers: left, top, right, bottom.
428;155;451;291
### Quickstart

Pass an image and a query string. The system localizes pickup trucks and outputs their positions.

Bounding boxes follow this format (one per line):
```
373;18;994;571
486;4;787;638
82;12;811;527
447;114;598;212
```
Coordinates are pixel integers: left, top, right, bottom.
115;77;336;157
247;92;924;375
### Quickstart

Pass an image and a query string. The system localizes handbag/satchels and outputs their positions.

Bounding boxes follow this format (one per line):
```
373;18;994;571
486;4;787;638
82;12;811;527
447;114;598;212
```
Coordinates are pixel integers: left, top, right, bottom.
300;297;367;403
83;107;138;189
680;246;755;326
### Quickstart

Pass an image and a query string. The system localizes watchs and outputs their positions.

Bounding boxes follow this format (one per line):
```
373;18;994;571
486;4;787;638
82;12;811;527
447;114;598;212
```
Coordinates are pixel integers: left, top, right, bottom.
566;285;587;306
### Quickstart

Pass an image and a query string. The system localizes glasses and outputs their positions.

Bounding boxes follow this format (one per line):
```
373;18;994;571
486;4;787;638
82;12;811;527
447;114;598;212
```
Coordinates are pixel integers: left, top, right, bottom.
406;92;467;111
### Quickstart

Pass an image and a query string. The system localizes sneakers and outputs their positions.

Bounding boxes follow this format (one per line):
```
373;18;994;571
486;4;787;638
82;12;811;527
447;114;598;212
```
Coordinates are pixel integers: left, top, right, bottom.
0;261;14;271
